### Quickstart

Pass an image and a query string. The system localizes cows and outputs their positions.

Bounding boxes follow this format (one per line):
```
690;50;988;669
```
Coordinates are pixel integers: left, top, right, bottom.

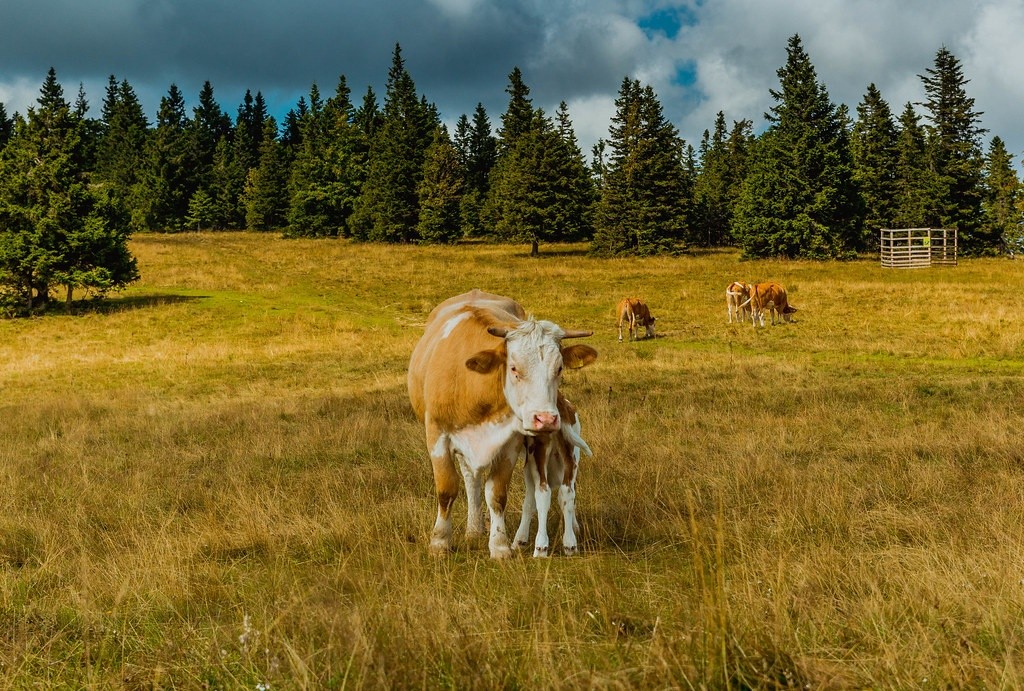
725;281;798;328
616;297;657;342
407;285;597;556
